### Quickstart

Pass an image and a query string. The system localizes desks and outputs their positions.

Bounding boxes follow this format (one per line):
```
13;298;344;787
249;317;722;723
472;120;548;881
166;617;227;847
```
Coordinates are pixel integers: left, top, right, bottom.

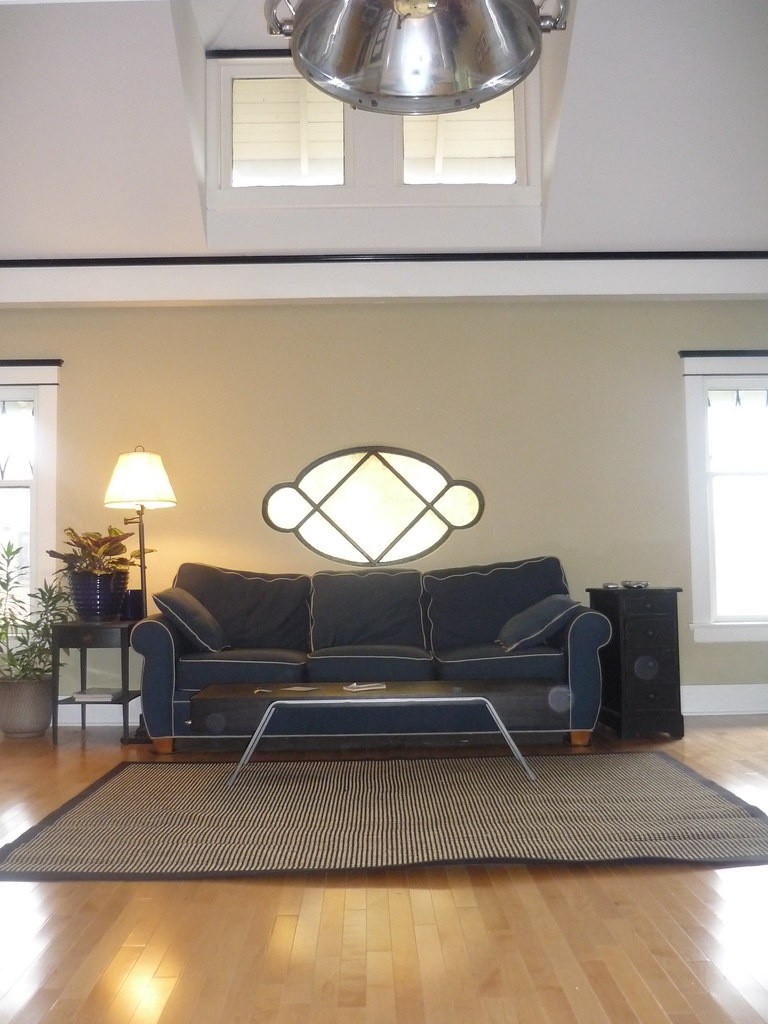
52;620;142;744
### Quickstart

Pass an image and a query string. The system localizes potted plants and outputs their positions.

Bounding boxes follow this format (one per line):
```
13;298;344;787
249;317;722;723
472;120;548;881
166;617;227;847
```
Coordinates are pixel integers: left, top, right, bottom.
0;540;79;738
46;526;158;622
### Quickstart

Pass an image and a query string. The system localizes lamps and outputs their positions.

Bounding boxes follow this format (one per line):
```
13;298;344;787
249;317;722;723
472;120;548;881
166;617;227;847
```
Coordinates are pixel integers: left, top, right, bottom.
263;0;570;117
104;445;176;745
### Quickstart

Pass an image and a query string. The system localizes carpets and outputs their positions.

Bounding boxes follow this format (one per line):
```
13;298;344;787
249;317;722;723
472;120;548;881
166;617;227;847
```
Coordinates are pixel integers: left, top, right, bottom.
0;750;768;882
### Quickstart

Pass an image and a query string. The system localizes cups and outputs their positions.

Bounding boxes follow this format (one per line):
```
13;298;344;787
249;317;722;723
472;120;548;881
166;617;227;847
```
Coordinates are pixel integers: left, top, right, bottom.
123;589;142;619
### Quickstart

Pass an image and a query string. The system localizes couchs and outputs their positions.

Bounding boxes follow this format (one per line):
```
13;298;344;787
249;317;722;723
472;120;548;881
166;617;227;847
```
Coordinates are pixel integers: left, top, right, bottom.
130;555;613;755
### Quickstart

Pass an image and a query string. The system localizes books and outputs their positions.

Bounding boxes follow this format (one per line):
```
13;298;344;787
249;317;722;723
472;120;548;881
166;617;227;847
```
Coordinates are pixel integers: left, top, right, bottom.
343;682;386;692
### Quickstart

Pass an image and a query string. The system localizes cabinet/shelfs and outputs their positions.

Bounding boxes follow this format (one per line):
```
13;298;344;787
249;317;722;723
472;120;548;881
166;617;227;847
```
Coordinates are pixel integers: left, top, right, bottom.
586;587;686;741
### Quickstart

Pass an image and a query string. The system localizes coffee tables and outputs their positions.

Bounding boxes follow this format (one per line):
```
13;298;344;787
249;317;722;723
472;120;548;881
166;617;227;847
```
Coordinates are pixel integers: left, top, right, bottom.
189;678;574;786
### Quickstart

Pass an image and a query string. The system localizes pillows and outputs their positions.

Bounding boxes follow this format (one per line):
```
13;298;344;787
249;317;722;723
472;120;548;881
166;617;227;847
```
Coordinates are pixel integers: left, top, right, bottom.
152;589;233;655
495;593;583;652
